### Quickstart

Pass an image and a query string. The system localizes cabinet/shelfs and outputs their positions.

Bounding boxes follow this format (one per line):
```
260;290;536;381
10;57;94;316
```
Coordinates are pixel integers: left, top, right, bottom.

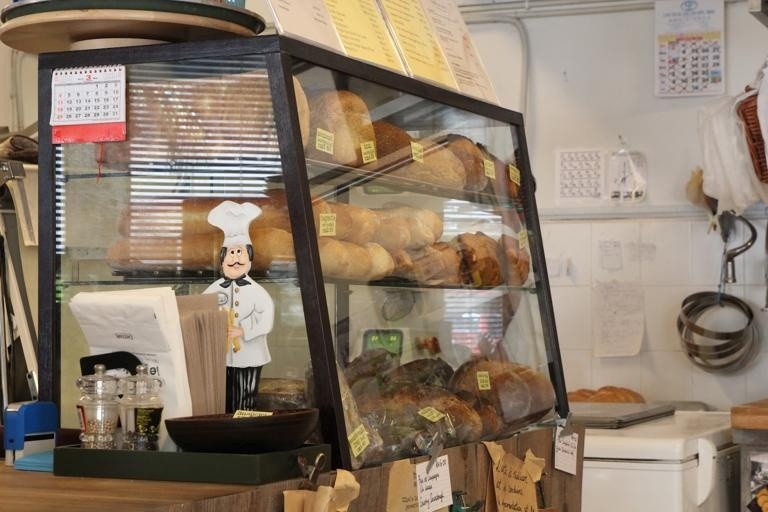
38;36;570;475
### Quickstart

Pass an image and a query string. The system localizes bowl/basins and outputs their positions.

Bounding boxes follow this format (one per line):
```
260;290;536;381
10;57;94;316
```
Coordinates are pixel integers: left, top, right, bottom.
164;407;322;455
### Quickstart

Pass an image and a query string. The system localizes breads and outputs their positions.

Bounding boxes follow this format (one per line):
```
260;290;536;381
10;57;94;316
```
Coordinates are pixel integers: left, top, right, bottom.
255;349;557;446
566;386;644;406
105;187;530;289
93;67;520;206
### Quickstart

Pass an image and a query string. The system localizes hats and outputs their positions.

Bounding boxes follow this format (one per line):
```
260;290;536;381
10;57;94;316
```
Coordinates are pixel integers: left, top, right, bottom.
208;200;262;248
